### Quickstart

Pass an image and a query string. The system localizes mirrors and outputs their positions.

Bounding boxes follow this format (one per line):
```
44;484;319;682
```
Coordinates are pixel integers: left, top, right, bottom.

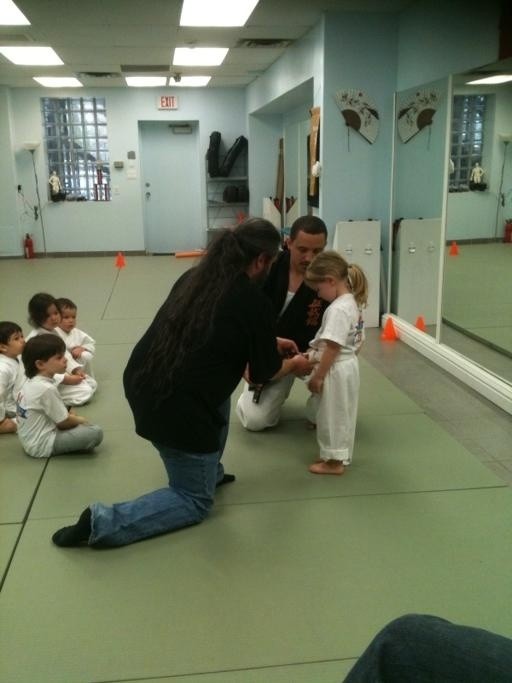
388;58;512;388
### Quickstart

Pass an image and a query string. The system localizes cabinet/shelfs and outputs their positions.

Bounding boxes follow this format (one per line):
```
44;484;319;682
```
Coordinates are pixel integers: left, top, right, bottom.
204;155;250;250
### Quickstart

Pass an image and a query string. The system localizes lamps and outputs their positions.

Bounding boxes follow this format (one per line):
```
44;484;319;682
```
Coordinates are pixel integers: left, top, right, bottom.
21;139;50;257
494;127;512;240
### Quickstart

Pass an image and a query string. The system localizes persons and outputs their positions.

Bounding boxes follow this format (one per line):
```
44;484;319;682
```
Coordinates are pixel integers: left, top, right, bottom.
235;216;331;432
52;217;314;548
303;251;369;475
0;293;102;459
343;614;512;682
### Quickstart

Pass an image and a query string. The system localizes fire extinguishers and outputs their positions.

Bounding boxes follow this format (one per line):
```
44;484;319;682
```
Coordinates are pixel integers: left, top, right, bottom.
504;218;512;242
24;233;33;259
